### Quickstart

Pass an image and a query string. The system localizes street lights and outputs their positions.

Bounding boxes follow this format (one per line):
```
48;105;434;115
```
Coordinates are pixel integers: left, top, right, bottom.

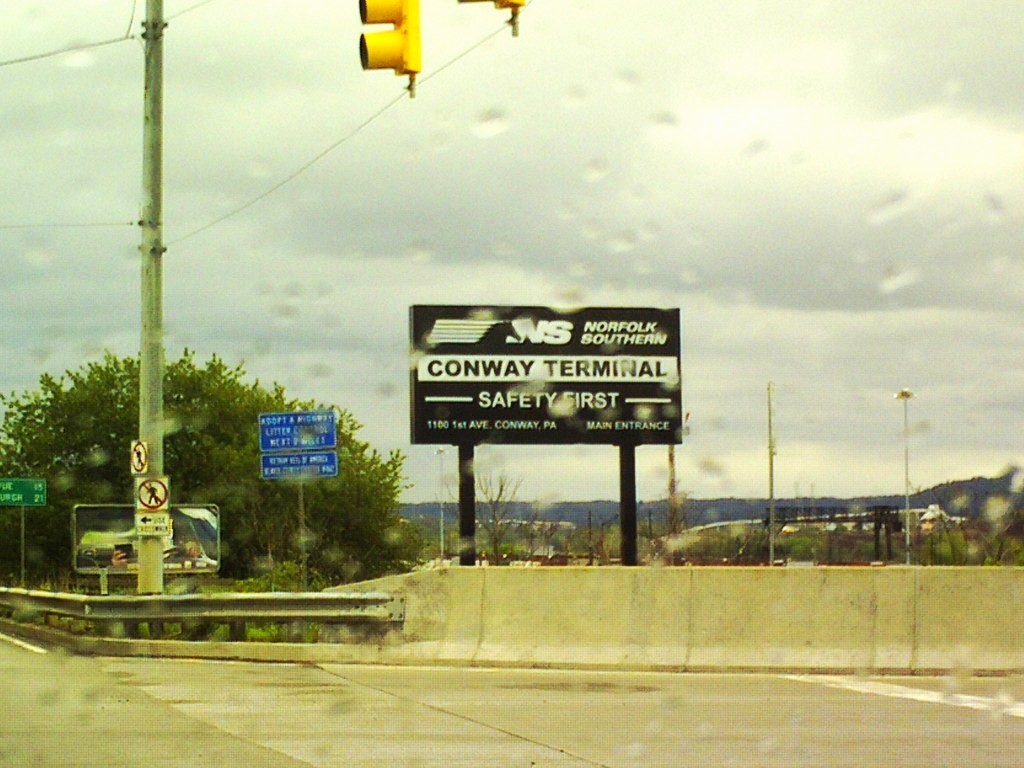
435;448;446;562
894;388;917;565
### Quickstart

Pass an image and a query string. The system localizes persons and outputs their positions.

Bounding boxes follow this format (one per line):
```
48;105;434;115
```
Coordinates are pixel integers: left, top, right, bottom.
111;508;205;563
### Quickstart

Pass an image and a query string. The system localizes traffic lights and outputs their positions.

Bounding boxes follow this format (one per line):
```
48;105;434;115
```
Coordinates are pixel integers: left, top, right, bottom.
358;0;422;75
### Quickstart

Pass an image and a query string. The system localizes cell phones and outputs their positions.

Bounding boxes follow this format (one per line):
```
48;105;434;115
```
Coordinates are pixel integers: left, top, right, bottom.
115;543;135;559
